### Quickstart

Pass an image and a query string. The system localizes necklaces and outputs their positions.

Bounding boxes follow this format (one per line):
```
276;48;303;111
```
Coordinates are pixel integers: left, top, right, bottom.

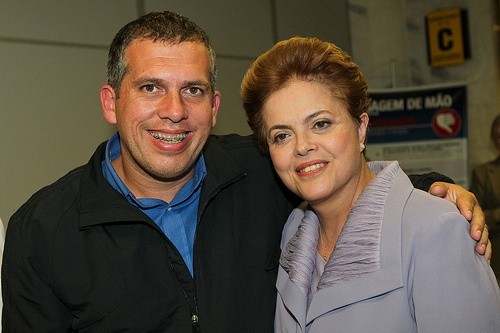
320;223;331;260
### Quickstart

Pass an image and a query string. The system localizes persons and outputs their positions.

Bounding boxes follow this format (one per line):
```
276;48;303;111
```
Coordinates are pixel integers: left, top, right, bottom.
2;9;492;333
240;36;499;332
